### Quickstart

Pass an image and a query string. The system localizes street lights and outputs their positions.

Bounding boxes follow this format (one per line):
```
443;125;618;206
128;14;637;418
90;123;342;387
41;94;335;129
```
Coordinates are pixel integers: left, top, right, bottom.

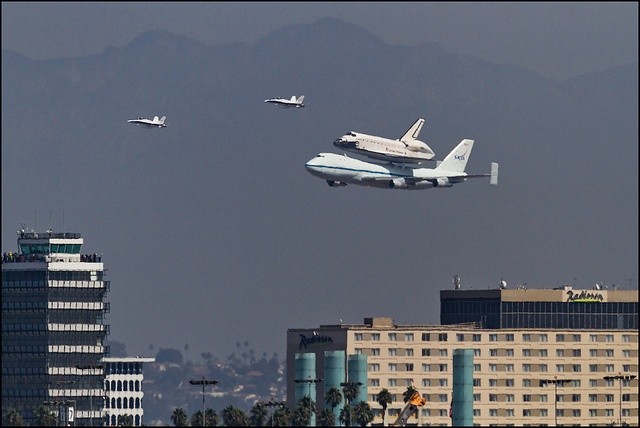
540;376;572;428
43;400;75;426
295;376;324;428
603;372;637;428
340;382;363;428
189;377;218;428
77;365;104;426
261;398;283;427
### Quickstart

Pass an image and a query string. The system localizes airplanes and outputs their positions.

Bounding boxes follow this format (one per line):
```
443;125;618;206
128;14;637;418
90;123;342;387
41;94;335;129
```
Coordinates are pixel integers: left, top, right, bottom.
305;139;498;189
264;96;304;108
127;116;166;128
333;118;438;165
